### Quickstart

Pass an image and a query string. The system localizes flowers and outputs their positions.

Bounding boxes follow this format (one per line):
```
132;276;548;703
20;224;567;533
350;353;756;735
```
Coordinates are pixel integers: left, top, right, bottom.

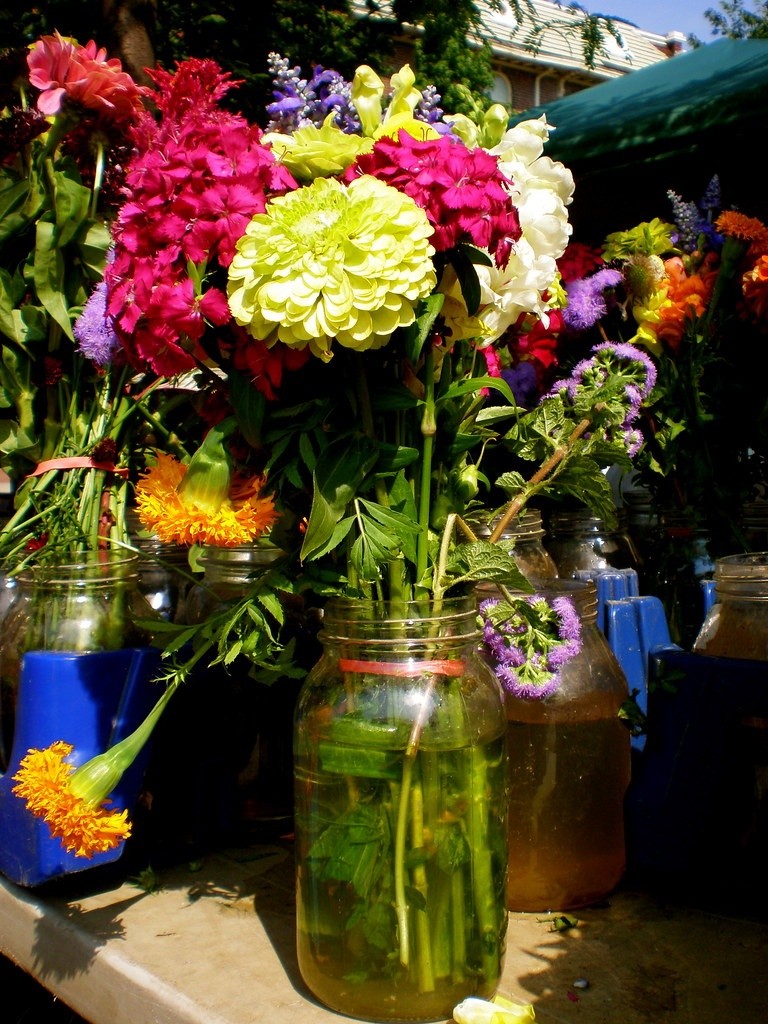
0;29;768;992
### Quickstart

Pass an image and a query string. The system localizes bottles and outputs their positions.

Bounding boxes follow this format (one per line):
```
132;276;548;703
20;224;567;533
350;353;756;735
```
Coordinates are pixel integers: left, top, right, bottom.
691;552;768;661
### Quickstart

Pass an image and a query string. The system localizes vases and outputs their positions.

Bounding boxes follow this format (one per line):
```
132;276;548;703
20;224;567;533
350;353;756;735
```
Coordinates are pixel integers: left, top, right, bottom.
131;536;205;624
576;509;643;569
186;545;285;625
548;517;608;578
0;548;165;774
456;507;560;578
689;551;768;659
507;577;631;912
291;594;507;1024
